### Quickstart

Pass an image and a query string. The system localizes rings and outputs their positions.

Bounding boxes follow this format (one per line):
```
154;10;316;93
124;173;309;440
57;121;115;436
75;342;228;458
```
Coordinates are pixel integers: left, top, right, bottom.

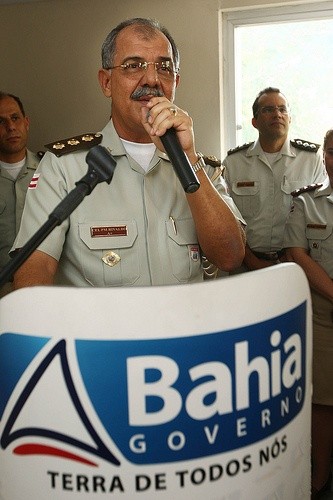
167;107;177;117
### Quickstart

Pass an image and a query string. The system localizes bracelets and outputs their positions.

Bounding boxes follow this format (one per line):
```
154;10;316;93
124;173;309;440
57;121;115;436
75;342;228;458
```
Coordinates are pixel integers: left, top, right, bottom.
192;152;206;173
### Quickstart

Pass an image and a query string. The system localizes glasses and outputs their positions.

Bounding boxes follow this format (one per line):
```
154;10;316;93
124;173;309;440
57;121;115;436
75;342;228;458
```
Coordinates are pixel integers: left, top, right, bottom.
255;106;289;117
106;60;177;78
323;149;333;156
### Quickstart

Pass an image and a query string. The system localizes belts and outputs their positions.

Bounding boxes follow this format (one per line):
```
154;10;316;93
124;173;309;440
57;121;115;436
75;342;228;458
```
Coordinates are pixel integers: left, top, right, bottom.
250;248;284;261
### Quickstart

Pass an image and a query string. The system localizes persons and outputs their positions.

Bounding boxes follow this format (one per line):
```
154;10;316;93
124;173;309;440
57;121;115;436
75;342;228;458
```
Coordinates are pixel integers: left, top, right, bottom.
0;91;46;297
220;88;328;276
278;130;333;500
8;18;247;291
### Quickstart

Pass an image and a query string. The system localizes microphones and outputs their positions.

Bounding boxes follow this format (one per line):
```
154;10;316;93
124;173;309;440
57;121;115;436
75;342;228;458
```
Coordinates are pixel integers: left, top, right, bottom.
145;109;200;193
